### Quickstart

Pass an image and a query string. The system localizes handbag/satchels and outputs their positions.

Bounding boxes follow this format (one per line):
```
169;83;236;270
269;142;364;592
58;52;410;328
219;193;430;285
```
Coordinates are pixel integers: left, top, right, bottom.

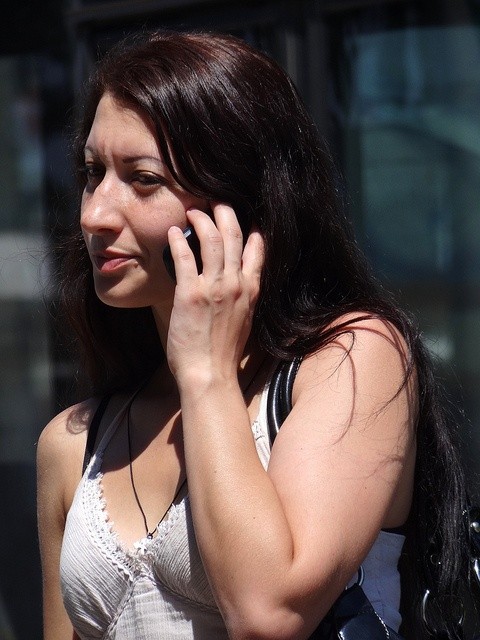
311;508;480;640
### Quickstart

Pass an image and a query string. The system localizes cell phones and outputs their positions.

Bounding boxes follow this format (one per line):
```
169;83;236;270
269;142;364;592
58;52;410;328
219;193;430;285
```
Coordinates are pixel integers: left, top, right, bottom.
163;226;202;285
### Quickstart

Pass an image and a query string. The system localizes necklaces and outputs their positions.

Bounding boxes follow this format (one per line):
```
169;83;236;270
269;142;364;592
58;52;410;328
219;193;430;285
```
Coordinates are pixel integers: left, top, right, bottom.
127;353;270;539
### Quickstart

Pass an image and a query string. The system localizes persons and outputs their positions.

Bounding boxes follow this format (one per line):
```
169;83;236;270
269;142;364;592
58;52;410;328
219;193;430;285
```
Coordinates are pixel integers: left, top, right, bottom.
35;28;478;639
